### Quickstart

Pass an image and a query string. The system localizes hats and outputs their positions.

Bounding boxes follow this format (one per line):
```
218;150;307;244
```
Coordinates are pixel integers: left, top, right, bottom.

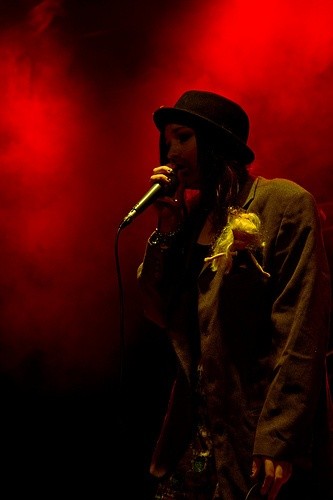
153;90;255;165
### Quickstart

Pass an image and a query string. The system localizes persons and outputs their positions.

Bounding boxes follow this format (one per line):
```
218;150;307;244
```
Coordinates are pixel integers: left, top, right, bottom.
132;89;331;499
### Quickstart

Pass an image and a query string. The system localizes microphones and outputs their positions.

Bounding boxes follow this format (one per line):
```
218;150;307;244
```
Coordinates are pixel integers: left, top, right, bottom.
121;163;177;226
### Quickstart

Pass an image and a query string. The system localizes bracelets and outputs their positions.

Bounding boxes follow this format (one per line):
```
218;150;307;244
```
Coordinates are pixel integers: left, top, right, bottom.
150;225;181;250
